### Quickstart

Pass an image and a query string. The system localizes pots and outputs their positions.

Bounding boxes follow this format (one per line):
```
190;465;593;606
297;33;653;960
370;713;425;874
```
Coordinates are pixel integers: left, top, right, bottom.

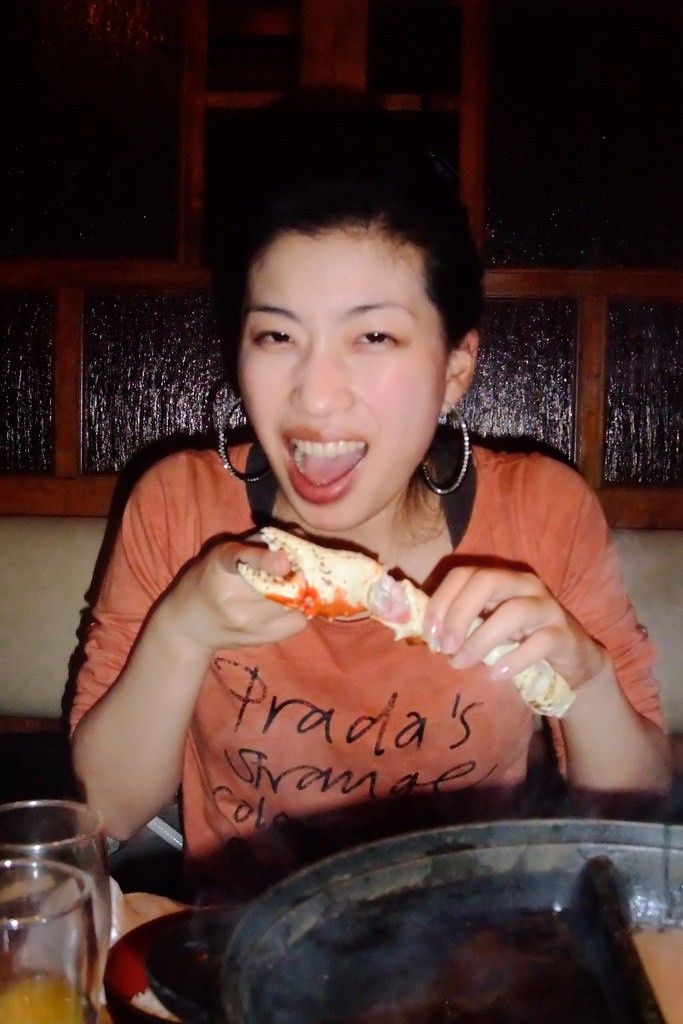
222;820;683;1023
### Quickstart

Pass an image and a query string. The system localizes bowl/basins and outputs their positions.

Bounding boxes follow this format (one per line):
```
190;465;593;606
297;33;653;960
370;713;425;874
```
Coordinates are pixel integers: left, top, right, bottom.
103;907;233;1024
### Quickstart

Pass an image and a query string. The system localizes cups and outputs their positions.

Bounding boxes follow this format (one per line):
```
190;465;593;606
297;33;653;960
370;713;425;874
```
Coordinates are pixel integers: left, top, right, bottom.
1;799;113;997
0;857;98;1024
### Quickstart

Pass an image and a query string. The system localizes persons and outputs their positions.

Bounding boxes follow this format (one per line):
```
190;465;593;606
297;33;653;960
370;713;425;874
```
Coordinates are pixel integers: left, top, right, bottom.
67;90;673;912
